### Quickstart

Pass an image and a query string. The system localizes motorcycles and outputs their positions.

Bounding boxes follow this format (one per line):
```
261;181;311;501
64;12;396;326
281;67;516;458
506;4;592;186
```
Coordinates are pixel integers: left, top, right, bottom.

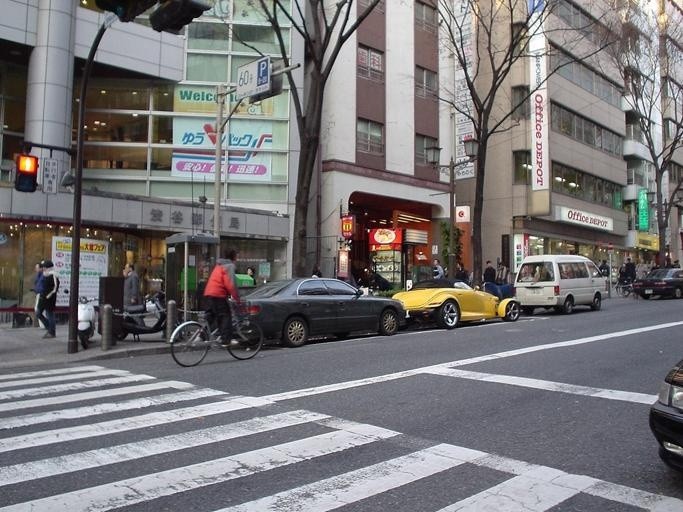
96;289;184;345
76;294;98;353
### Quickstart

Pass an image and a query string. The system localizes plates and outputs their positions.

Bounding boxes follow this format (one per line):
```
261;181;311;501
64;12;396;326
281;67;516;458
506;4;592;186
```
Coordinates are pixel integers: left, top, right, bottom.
373;229;396;244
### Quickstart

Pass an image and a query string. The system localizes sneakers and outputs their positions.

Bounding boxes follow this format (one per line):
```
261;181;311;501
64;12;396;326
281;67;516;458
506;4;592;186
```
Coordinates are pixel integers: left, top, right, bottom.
221;339;239;347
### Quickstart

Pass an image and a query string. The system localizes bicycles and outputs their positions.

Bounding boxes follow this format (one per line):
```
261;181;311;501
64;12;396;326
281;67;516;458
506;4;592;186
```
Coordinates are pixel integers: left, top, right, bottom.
165;297;263;369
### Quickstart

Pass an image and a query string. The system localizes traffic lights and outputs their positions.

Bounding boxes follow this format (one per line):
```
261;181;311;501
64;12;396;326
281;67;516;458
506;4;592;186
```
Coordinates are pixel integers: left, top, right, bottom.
148;0;213;36
12;151;40;193
93;0;157;25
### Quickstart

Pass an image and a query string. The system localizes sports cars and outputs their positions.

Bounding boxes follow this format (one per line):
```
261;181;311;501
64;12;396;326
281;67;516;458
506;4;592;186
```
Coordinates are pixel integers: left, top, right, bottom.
393;277;524;330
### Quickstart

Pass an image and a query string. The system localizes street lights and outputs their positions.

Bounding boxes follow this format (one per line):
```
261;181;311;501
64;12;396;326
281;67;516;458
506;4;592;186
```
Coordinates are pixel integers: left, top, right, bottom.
423;132;480;283
645;186;682;217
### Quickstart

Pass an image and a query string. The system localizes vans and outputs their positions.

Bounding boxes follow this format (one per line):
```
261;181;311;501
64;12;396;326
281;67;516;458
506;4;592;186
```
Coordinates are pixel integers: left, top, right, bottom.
513;252;607;316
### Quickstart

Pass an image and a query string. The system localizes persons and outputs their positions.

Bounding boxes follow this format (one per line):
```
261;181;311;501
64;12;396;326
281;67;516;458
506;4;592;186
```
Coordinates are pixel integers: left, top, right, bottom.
123;263;142;314
431;259;511;286
651;256;681;272
246;265;256;285
312;263;322;278
34;261;60;338
598;258;636;284
32;262;46;328
193;248;241;348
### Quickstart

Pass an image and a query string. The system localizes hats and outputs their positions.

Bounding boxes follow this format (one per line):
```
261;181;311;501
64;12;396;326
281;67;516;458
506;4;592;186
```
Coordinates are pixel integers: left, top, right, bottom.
38;260;54;269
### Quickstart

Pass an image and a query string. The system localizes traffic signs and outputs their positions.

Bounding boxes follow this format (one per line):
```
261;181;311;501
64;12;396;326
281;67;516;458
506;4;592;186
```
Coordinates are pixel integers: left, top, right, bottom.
606;242;613;251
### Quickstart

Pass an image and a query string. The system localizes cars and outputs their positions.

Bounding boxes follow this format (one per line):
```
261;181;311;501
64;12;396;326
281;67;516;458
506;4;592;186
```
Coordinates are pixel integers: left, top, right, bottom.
644;356;682;474
227;272;405;349
630;266;682;300
